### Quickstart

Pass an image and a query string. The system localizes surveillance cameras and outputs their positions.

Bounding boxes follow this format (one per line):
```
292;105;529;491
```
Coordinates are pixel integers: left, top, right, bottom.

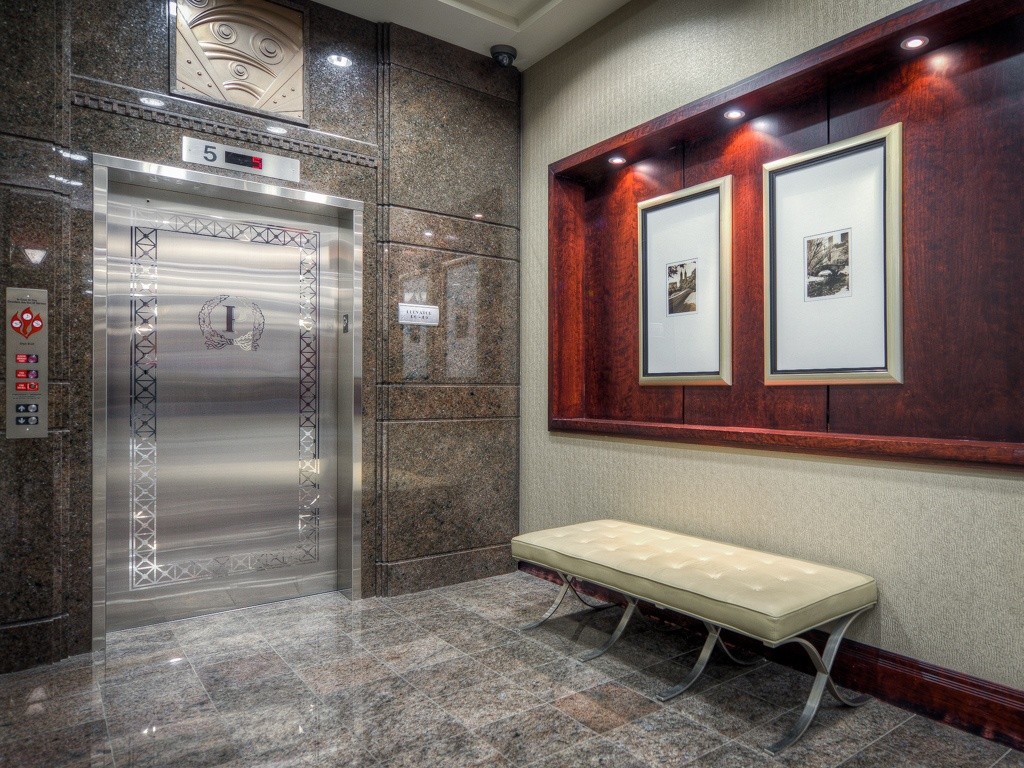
489;44;517;67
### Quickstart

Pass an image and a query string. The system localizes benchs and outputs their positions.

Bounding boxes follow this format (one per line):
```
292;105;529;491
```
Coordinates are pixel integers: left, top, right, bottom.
511;518;881;758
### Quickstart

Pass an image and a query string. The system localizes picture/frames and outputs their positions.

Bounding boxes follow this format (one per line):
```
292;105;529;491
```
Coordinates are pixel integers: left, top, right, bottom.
761;122;906;386
636;173;733;387
